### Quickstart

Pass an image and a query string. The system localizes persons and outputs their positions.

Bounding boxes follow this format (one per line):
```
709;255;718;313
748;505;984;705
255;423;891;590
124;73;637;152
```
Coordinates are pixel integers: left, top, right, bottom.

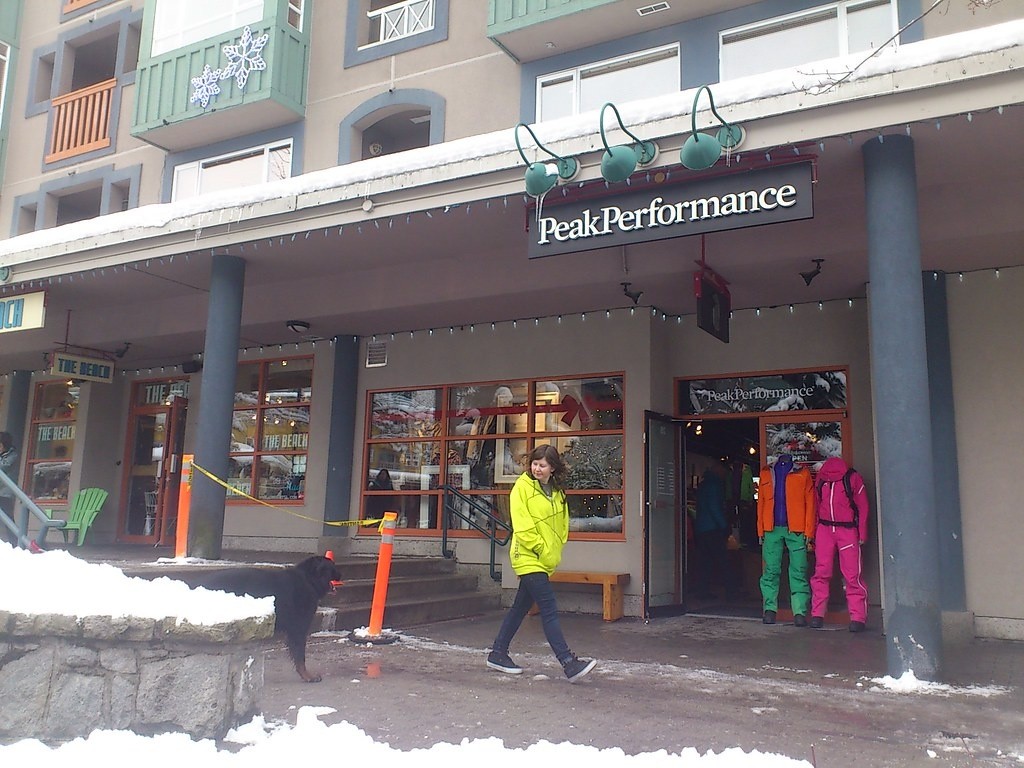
810;458;869;631
484;444;598;683
368;469;393;516
0;431;18;549
687;464;739;609
756;454;815;626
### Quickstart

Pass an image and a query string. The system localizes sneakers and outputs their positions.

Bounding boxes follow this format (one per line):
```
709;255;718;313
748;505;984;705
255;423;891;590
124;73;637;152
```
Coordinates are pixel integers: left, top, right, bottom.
849;622;864;632
486;651;522;674
795;614;807;626
564;660;598;683
810;616;824;627
763;610;776;624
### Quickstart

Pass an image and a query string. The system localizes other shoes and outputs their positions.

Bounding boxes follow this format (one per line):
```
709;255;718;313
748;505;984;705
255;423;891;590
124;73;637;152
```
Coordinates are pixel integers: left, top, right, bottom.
728;589;759;602
694;591;718;600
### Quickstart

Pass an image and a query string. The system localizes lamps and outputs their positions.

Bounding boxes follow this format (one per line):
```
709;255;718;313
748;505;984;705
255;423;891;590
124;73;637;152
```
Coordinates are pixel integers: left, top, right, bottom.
680;85;746;171
515;121;581;198
600;102;659;184
287;321;310;333
182;360;202;374
621;282;644;305
799;259;825;284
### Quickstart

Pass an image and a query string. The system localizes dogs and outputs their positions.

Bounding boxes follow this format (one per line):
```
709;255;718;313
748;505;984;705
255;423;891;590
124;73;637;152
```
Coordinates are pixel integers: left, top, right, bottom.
185;556;341;683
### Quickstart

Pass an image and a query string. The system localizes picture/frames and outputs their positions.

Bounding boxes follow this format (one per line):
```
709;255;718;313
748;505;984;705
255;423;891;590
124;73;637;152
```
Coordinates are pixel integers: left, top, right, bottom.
494;391;559;483
419;465;470;530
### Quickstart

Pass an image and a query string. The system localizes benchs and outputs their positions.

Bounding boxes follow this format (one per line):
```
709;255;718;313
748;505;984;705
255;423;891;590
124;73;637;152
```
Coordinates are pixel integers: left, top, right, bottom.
517;571;630;622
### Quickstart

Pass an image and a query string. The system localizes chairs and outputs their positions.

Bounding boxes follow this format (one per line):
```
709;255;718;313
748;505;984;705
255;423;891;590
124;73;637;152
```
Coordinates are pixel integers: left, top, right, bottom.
46;487;108;547
